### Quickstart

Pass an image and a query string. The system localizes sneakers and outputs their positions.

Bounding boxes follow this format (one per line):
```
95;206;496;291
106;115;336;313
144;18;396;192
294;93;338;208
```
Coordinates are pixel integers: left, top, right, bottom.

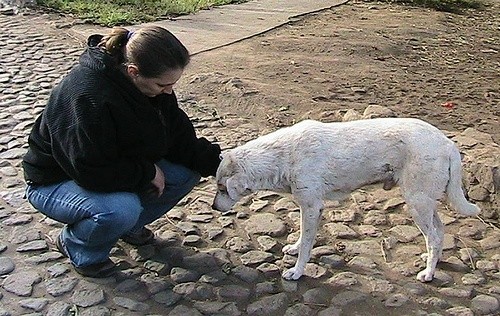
120;226;155;246
55;231;119;278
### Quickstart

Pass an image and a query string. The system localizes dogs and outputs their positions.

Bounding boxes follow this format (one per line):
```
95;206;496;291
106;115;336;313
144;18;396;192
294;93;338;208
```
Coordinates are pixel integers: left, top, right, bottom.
212;117;481;284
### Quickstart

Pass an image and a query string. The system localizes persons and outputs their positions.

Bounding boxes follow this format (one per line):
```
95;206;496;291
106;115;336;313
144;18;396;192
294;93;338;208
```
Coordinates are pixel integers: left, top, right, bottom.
21;25;221;282
